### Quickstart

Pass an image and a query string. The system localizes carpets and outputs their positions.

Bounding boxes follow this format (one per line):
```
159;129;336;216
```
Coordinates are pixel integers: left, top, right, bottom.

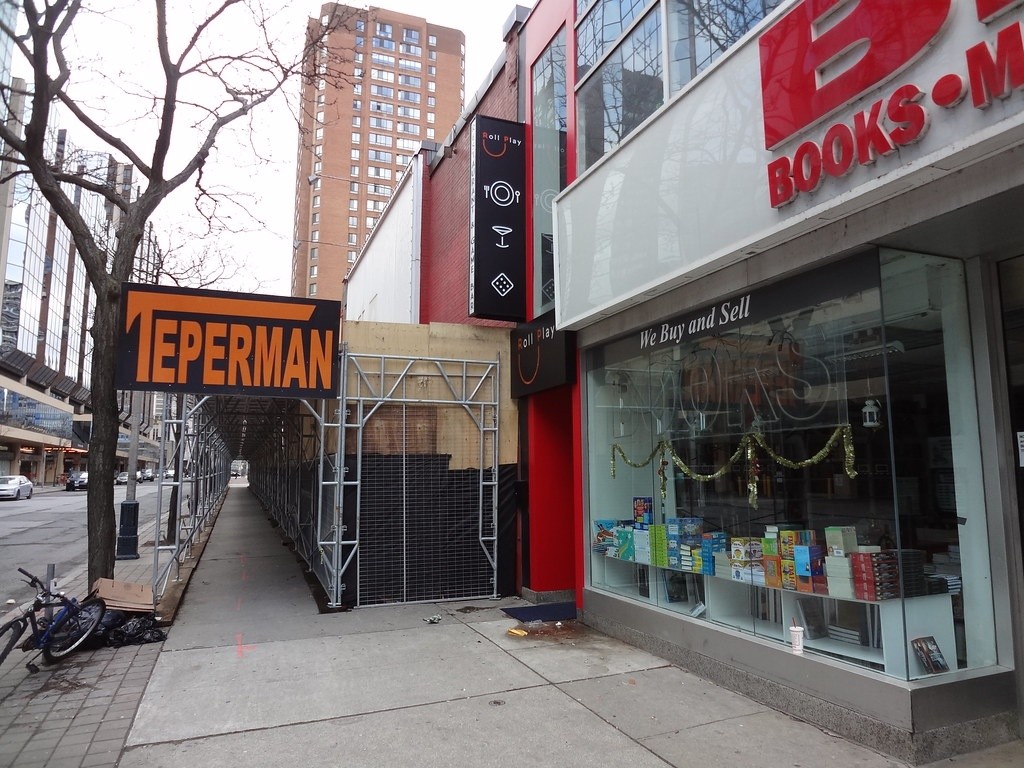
499;601;577;623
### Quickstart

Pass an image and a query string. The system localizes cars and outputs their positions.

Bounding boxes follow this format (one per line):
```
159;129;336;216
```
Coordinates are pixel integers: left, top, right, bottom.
116;472;128;485
231;471;241;476
165;470;174;480
0;475;33;501
137;471;144;485
66;471;88;491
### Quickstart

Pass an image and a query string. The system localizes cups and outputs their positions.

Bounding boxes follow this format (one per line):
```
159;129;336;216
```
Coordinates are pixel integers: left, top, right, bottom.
790;626;804;653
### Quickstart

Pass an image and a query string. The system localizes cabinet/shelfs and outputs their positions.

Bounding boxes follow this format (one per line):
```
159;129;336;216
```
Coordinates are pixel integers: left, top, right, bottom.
591;552;959;679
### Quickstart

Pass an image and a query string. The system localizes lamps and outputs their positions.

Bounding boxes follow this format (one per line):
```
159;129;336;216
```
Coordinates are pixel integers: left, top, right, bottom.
723;365;779;384
861;370;880;427
294;239;361;249
308;174;393;190
825;330;905;366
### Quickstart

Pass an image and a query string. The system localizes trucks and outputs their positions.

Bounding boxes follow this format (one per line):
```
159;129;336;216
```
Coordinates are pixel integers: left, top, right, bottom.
142;469;155;482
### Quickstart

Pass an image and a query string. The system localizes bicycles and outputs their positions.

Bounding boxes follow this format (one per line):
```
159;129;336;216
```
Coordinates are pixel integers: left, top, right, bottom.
0;567;107;674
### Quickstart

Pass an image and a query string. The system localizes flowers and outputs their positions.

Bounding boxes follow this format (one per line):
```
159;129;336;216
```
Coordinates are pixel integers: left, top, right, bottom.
611;425;858;510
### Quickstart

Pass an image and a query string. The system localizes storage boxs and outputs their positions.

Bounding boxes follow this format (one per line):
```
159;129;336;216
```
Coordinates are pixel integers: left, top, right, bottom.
691;526;926;601
593;497;703;569
